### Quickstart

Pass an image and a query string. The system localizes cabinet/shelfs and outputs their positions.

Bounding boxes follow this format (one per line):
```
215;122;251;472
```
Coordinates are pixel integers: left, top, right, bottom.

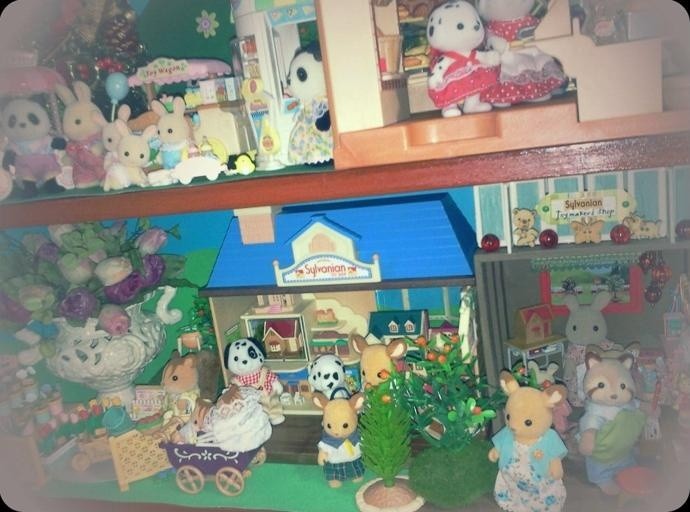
3;0;690;512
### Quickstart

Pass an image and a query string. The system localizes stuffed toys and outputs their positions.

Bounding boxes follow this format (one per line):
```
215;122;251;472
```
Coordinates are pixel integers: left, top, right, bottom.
307;336;408;486
427;0;568;117
486;291;647;511
158;338;282;452
0;0;330;196
